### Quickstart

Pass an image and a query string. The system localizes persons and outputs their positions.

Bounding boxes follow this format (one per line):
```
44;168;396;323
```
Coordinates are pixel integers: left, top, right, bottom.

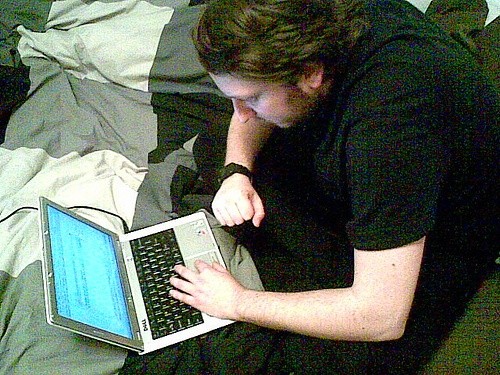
168;1;500;375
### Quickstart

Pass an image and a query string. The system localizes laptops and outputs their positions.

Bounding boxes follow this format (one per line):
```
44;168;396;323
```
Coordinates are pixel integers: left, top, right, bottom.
38;195;238;355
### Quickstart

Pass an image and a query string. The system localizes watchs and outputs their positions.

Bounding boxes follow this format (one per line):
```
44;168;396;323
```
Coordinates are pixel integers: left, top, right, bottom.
219;163;251;179
